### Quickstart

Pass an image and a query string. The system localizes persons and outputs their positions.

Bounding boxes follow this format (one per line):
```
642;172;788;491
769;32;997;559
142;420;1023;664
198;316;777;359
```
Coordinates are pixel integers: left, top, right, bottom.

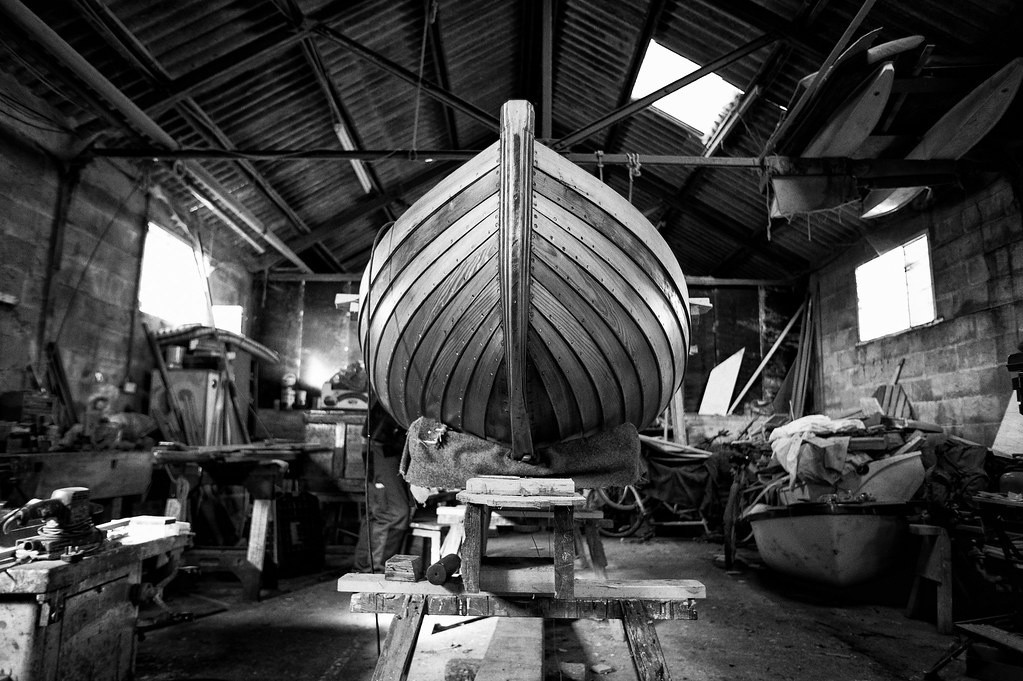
351;398;414;573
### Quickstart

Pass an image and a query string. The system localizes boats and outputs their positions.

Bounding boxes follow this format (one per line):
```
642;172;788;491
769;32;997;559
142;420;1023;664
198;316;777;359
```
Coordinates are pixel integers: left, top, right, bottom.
739;460;921;597
333;99;712;462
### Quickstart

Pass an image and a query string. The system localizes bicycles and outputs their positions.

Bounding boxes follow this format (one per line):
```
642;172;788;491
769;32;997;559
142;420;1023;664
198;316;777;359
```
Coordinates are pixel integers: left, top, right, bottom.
585;412;773;568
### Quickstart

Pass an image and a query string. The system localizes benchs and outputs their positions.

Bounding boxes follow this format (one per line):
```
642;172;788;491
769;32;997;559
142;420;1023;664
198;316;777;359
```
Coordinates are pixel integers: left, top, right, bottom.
436;506;609;569
335;572;706;681
